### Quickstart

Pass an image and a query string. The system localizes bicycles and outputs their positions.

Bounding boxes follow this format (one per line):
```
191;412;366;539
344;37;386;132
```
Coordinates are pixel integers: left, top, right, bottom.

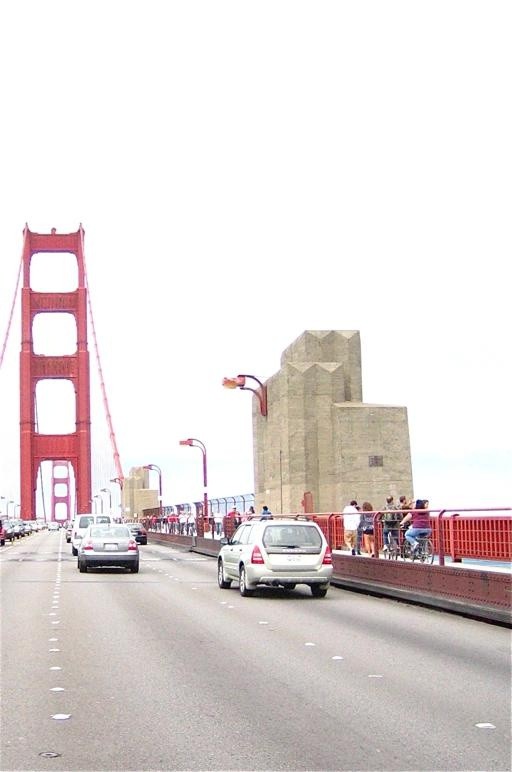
376;518;400;560
400;521;435;565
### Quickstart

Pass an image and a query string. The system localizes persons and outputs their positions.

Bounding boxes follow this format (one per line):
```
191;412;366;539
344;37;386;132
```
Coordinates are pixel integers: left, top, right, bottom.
137;505;273;537
342;493;434;564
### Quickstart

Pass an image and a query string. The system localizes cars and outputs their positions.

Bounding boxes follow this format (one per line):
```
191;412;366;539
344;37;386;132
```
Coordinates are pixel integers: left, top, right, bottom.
1;513;75;547
77;523;140;574
125;522;148;545
216;513;335;598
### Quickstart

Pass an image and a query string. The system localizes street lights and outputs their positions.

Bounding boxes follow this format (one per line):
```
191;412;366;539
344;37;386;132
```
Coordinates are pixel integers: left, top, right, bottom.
179;438;209;532
143;464;164;533
80;478;124;520
6;500;14;520
14;504;21;518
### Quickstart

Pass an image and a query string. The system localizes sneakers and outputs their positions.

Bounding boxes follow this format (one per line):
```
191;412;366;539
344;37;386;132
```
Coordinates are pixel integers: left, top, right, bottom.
383;545;388;552
352;548;355;555
411;541;419;552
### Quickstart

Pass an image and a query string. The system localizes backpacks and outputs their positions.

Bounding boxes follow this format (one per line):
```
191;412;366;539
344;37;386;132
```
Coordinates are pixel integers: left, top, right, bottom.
363;513;374;534
385;506;397;527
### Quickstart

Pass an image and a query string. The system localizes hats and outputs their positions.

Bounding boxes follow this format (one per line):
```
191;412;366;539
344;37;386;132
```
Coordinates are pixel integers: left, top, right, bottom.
232;506;236;509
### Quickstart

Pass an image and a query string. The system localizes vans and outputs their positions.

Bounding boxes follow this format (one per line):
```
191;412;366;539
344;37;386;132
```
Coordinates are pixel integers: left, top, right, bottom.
71;513;112;556
